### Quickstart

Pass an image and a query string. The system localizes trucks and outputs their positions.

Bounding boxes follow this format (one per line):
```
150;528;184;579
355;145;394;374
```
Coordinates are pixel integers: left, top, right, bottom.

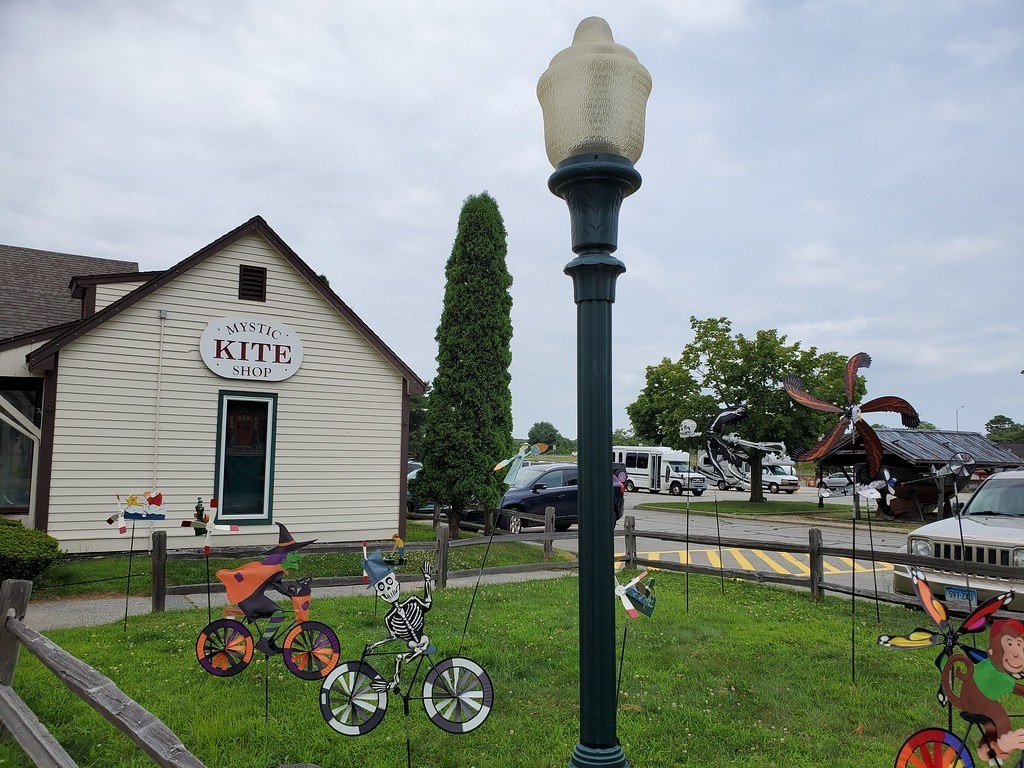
612;446;708;496
697;450;800;494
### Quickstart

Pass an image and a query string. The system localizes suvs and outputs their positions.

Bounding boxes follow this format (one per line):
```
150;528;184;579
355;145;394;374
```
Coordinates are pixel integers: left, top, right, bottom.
612;463;628;489
892;470;1024;612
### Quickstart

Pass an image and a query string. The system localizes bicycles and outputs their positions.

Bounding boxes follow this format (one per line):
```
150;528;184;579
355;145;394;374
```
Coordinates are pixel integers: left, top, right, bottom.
196;576;341;681
320;644;494;736
894;712;1024;768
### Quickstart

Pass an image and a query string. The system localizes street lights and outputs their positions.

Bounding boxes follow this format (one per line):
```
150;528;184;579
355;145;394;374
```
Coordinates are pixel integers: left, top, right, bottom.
956;406;964;431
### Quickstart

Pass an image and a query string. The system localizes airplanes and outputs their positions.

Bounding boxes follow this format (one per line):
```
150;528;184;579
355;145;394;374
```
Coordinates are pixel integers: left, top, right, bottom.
818;468;897;516
614;571;656;619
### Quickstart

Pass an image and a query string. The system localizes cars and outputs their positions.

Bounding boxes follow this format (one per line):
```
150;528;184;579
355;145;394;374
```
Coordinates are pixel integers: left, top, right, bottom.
407;461;448;520
536;15;652;768
816;472;856;489
446;464;625;534
